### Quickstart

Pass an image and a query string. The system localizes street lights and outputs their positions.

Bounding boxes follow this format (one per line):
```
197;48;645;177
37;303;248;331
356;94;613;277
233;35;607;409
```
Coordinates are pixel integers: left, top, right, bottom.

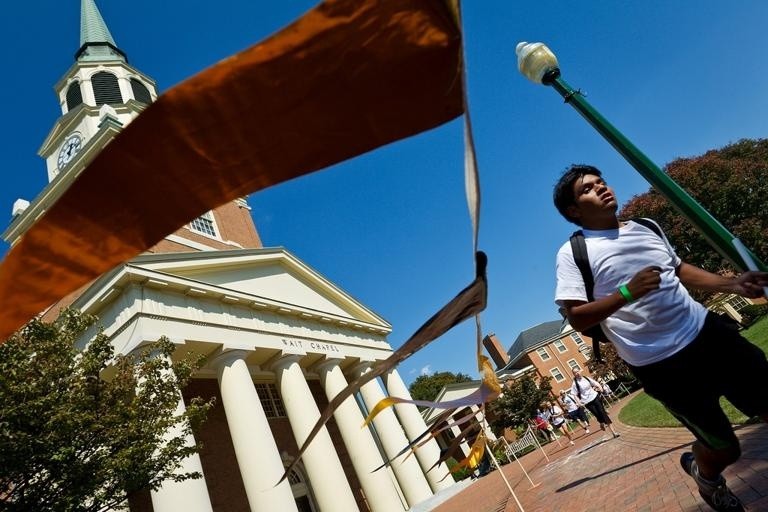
513;36;767;299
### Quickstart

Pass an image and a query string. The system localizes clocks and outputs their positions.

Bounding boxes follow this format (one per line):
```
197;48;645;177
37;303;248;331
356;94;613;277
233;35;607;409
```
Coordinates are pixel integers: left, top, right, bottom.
57;135;82;171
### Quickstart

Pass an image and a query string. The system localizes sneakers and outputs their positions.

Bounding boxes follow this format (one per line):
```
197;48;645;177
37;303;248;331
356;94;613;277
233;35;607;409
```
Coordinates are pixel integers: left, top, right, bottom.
569;441;574;446
585;429;589;434
614;433;620;438
600;423;605;431
680;452;746;512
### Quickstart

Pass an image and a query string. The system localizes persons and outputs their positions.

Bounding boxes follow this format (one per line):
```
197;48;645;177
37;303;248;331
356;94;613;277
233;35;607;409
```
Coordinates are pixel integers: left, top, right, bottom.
552;164;767;512
531;367;621;447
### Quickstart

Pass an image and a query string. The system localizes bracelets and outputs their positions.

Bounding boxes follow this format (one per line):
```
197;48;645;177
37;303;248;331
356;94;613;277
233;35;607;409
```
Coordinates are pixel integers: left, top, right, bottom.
616;285;633;303
